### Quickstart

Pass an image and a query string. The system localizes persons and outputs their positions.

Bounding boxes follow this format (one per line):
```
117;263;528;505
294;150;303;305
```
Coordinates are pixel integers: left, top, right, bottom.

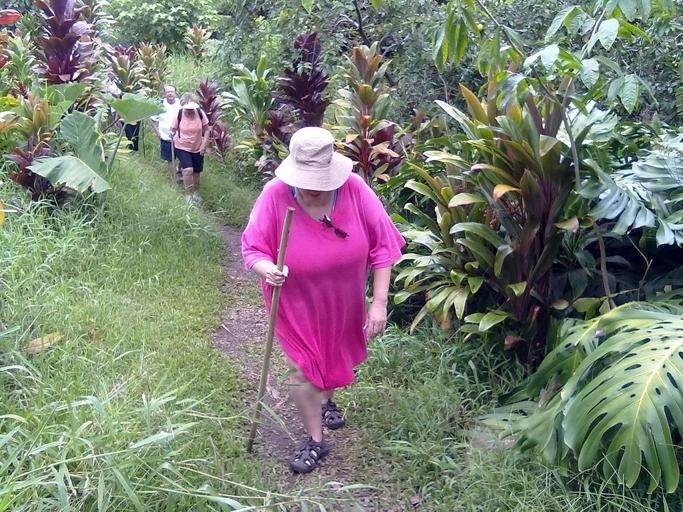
148;84;180;180
241;124;406;476
169;91;211;205
116;117;141;151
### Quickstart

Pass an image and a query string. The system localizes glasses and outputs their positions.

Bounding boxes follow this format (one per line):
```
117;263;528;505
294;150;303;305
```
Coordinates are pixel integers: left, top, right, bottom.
318;214;350;239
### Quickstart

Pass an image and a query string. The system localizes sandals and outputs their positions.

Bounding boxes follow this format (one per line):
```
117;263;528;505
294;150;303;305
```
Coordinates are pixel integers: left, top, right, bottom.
320;398;345;430
290;436;328;474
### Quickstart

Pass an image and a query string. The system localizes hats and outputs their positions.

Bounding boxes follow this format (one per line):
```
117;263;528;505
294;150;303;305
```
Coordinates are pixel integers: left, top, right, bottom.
182;101;201;109
275;126;353;192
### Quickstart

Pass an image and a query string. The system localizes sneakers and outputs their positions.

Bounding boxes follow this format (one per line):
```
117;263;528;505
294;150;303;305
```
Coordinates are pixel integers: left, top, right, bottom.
186;191;203;206
175;165;184;182
